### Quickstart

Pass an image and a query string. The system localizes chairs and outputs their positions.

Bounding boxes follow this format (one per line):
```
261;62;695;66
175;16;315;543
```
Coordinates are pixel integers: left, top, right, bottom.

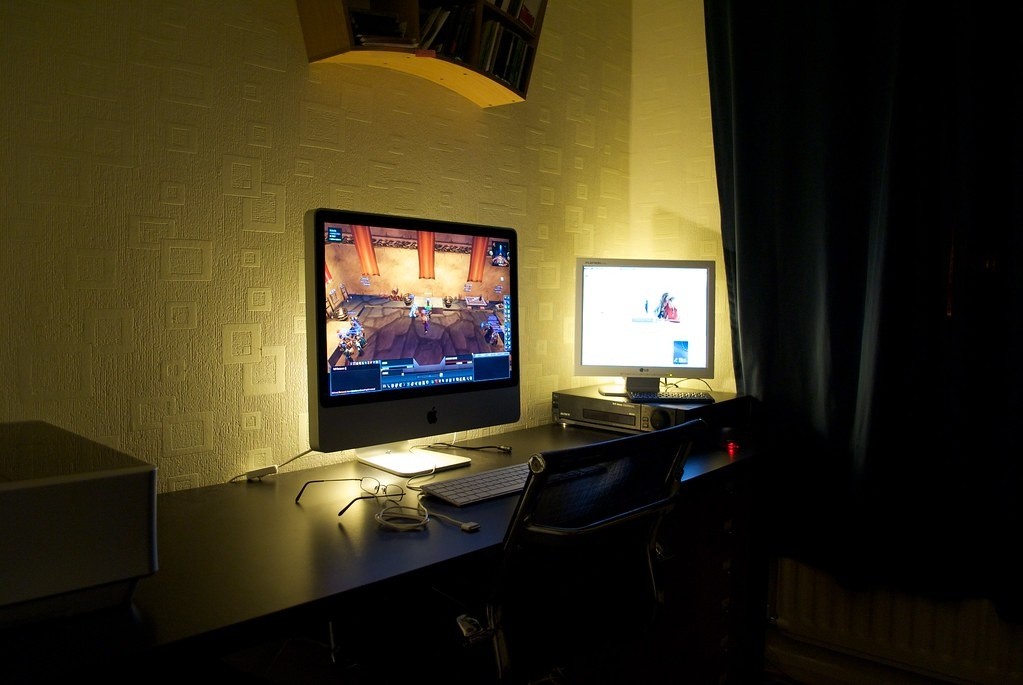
327;417;708;685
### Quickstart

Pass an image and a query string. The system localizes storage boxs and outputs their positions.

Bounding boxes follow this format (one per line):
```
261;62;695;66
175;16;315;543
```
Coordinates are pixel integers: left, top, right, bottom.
0;419;160;609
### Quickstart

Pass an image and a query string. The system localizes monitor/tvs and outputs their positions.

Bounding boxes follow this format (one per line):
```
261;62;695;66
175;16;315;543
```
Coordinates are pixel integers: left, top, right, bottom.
574;258;717;395
303;207;521;477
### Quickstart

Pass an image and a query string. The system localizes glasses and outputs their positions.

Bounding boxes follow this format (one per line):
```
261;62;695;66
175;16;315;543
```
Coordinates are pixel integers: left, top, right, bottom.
294;476;407;516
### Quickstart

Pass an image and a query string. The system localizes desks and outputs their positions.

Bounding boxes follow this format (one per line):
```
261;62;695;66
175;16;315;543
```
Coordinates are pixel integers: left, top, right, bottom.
0;424;746;685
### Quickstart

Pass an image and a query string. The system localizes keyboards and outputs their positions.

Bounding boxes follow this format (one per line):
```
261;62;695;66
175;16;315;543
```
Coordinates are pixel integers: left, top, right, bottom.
420;461;530;507
628;391;716;403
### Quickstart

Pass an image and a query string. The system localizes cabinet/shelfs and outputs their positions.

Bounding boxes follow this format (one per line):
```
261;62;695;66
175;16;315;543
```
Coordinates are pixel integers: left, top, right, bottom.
293;0;548;108
